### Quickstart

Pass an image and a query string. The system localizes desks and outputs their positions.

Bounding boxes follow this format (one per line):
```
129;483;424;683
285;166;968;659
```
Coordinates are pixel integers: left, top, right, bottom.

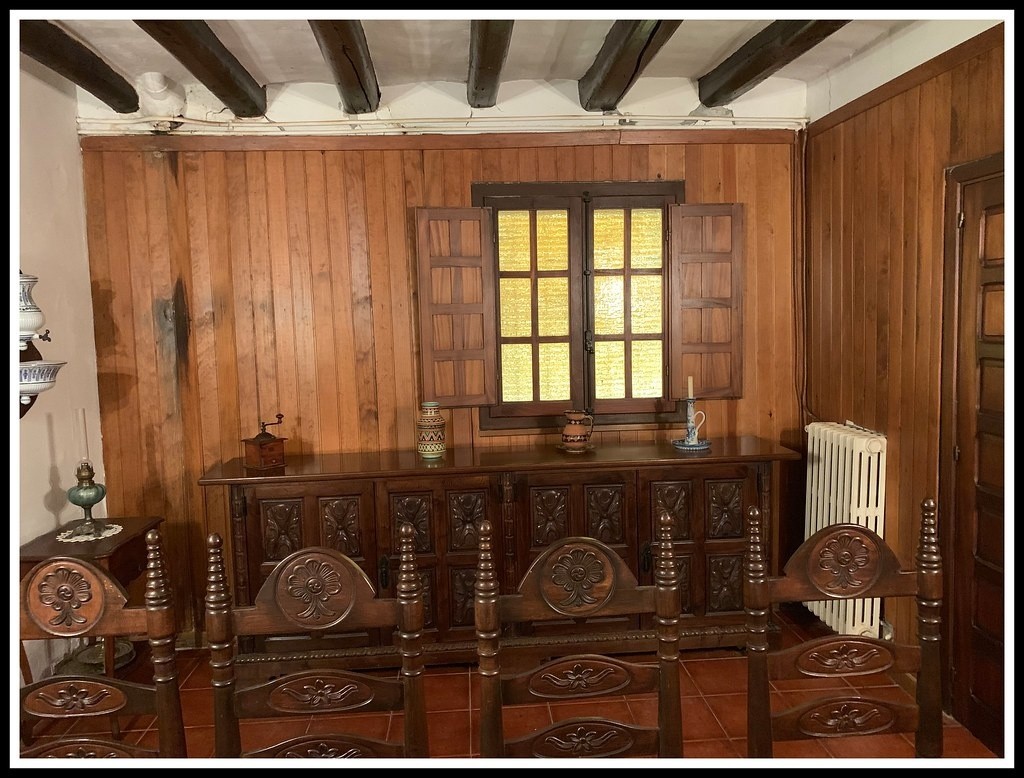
20;517;177;747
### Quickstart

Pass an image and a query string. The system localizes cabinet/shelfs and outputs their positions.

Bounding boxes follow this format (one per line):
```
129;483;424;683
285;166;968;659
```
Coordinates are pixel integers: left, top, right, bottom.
198;435;799;651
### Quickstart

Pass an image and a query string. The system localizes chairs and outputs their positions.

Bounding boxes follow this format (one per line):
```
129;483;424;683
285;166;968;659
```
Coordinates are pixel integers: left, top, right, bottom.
20;496;943;758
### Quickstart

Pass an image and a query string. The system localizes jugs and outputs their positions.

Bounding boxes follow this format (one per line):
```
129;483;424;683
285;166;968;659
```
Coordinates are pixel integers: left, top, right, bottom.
562;409;595;453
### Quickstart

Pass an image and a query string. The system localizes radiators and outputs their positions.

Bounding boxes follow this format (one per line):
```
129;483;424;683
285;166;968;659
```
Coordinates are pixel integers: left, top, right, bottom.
801;420;888;636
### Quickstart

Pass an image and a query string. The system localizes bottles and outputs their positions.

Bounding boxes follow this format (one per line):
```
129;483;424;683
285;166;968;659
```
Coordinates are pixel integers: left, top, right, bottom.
417;402;445;459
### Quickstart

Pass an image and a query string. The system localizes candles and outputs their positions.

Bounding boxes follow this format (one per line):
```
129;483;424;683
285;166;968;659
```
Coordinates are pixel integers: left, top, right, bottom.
687;376;694;399
78;408;89;461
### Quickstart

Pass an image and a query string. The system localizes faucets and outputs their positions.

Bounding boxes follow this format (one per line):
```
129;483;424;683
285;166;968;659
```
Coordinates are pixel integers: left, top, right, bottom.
38;329;52;343
